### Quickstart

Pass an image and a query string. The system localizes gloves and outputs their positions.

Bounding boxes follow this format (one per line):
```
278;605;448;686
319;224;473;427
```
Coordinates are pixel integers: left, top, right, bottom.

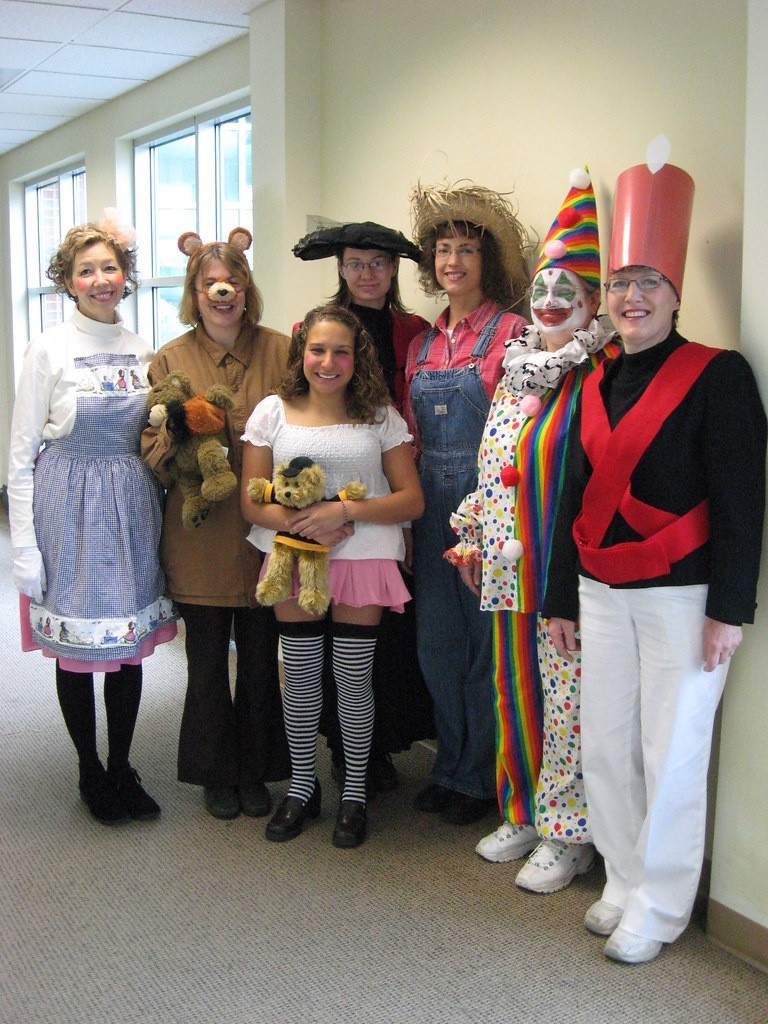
13;546;48;604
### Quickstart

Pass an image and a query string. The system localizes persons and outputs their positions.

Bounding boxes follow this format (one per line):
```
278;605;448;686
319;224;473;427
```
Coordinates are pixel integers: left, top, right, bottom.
441;269;619;895
7;222;182;825
241;305;424;848
291;220;429;800
403;188;535;826
139;227;294;820
543;265;768;963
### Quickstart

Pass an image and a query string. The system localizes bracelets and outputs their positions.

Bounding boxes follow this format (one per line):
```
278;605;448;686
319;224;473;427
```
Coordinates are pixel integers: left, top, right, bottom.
340;501;348;523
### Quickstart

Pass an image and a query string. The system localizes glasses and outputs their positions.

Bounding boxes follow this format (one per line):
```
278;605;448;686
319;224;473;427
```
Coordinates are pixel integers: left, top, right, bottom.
606;275;671;294
432;247;484;259
341;262;396;273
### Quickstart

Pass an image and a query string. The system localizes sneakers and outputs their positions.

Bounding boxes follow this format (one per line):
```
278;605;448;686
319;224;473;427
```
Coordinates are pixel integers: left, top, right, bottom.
603;925;663;963
514;838;597;893
584;896;627;935
475;821;545;862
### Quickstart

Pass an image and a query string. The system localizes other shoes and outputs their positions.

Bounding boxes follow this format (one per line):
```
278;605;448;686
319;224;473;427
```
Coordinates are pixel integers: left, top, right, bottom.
414;784;494;823
239;778;271;818
78;760;131;825
205;783;239;820
107;763;160;819
367;745;399;788
329;761;373;802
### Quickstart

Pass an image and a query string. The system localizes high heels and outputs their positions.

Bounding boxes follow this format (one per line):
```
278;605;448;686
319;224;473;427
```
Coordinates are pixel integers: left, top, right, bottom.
332;800;364;846
264;777;320;841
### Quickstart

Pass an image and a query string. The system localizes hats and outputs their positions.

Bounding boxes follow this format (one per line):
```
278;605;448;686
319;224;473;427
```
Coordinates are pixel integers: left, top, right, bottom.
607;135;695;303
531;166;601;290
292;219;425;265
410;184;532;304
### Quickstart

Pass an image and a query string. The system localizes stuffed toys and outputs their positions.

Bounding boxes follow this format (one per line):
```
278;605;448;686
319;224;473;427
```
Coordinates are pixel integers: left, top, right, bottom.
145;370;236;531
247;456;369;617
